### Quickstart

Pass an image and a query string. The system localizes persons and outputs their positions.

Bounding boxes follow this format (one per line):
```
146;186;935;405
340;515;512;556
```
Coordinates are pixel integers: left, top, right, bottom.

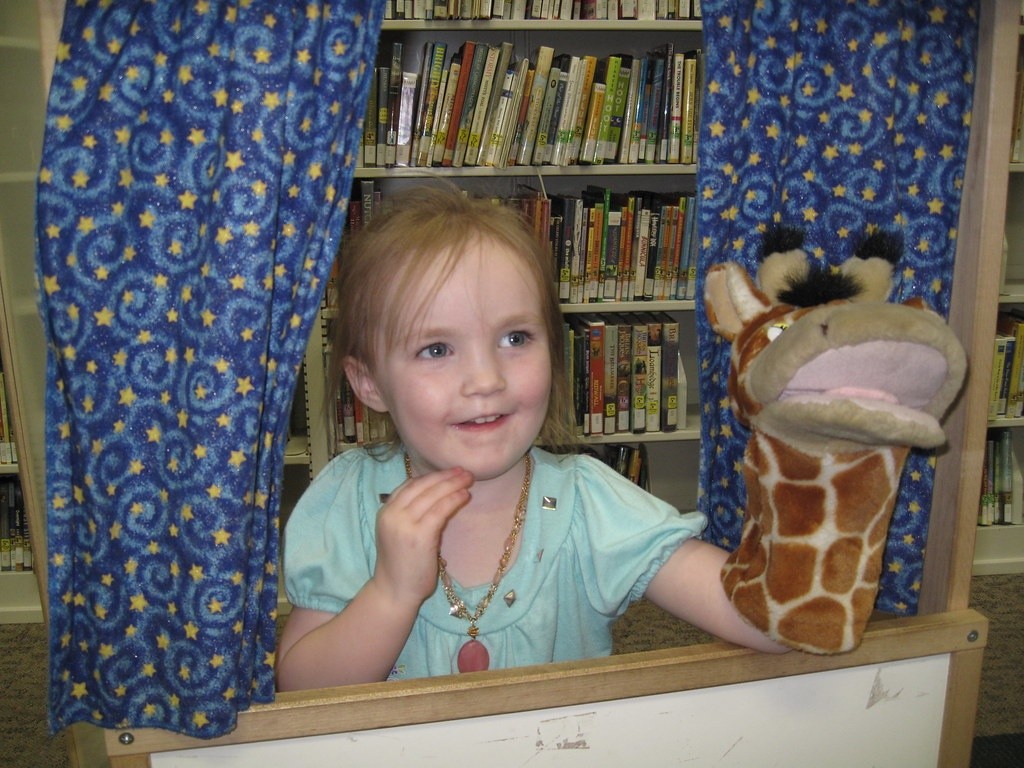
275;177;797;692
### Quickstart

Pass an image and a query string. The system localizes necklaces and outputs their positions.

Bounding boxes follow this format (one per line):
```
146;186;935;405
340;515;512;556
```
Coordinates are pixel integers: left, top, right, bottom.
402;449;534;676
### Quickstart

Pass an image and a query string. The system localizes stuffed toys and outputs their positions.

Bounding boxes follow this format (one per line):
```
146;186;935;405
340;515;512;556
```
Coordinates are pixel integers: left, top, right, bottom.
702;226;967;657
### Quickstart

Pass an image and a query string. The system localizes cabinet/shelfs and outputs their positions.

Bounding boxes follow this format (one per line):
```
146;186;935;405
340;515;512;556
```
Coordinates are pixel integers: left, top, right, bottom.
284;0;1024;577
0;283;49;625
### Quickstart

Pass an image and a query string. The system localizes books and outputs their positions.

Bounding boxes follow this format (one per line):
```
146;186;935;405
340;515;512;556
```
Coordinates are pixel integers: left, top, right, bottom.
0;318;38;576
313;180;701;514
974;36;1024;528
358;3;703;165
